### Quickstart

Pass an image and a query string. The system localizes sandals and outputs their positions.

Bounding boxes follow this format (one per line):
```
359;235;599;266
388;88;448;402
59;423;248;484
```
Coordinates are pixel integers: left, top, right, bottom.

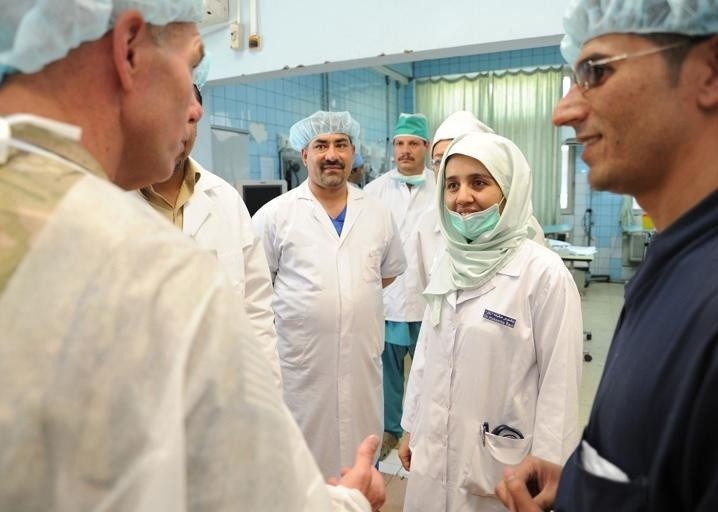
376;433;395;462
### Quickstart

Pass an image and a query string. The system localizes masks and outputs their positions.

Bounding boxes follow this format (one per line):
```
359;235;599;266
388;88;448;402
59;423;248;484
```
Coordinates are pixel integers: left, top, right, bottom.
388;175;427;186
444;196;507;241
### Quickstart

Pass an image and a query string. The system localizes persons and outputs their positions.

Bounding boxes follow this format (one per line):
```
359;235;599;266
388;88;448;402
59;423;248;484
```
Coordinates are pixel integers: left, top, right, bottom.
248;110;408;487
400;132;583;512
0;0;387;512
363;113;436;442
498;0;717;511
128;50;284;398
414;110;494;300
349;153;365;187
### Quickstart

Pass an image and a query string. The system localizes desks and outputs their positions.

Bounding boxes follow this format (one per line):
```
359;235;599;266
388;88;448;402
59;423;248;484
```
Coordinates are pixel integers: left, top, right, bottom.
563;254;593;288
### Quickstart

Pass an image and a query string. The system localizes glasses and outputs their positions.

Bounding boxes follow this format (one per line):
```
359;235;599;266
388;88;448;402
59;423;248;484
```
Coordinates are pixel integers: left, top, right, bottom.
571;36;696;89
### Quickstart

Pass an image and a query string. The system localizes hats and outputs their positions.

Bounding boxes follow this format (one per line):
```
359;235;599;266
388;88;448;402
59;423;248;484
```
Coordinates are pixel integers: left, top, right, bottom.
187;53;212;108
1;0;206;77
288;110;360;152
392;111;430;142
561;0;718;45
